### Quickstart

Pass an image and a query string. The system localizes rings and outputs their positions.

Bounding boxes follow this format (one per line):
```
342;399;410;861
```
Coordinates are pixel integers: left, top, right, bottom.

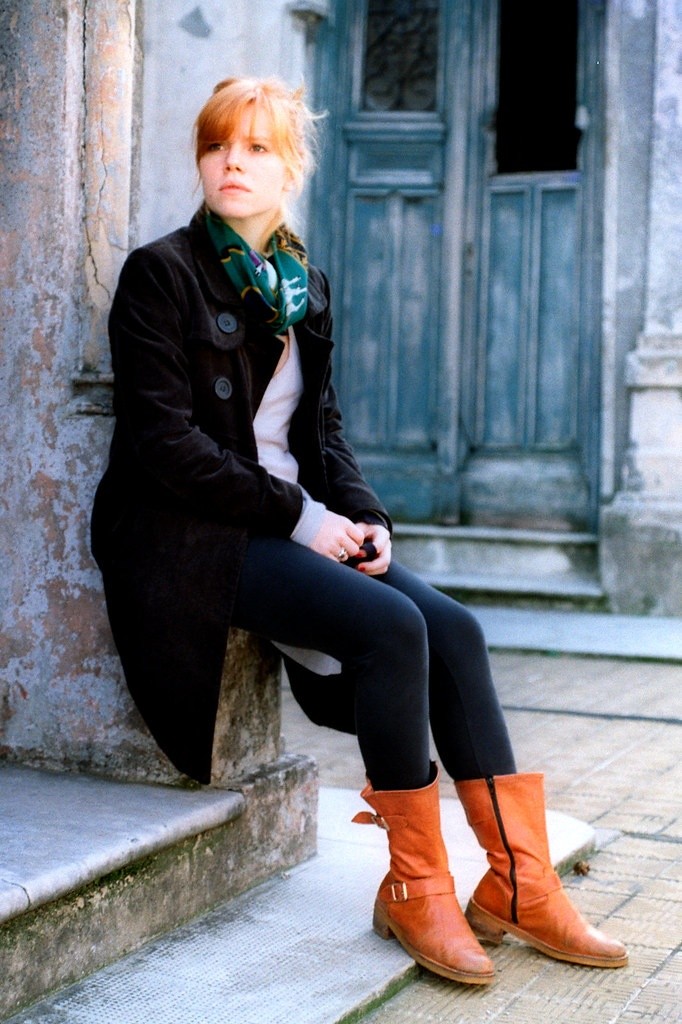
337;546;347;559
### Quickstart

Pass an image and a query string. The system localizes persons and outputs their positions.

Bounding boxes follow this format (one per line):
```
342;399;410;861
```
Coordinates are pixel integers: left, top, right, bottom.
91;77;630;985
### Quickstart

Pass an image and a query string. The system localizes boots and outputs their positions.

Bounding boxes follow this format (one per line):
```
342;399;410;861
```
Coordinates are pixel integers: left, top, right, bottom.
351;761;497;984
454;774;628;967
